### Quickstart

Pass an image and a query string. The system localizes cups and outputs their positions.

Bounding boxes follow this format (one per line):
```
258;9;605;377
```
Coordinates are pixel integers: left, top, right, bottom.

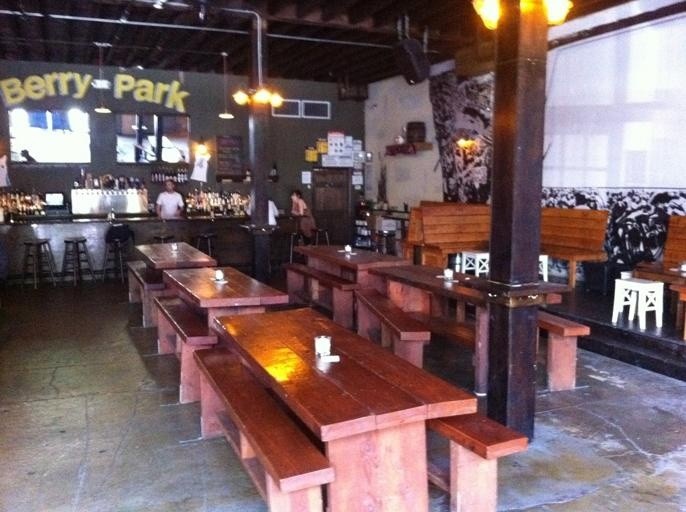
314;335;332;357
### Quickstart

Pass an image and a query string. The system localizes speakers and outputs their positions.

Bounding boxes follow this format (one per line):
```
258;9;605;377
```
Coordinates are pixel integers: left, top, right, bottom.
396;38;431;86
406;121;426;143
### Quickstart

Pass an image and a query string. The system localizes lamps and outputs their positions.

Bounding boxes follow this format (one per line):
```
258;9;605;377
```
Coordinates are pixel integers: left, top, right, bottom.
93;1;574;123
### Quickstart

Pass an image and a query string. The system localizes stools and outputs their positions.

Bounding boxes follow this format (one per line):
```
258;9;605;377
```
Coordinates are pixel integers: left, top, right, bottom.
198;233;218;259
22;239;58;289
59;236;97;289
153;232;175;244
312;228;330;246
286;230;303;263
612;277;665;331
102;230;134;286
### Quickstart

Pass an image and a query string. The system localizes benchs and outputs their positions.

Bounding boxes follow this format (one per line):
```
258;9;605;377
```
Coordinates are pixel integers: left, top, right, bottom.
154;294;219;404
540;206;610;288
353;288;430;369
283;262;361;329
425;413;529;512
419;203;492;268
126;260;166;328
192;346;336;512
456;299;590;392
399;208;422;260
632;214;685;328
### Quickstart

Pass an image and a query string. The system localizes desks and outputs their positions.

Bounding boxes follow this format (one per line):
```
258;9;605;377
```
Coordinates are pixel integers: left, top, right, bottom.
460;249;549;282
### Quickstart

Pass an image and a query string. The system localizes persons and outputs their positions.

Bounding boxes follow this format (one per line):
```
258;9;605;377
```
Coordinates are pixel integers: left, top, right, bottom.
243;194;280;227
154;177;185;220
287;189;318;248
0;130;13;193
